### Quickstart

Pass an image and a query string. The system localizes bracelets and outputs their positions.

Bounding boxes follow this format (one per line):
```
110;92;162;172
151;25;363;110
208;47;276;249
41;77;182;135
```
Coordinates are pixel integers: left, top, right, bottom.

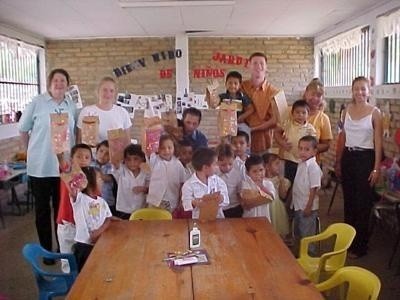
373;169;381;173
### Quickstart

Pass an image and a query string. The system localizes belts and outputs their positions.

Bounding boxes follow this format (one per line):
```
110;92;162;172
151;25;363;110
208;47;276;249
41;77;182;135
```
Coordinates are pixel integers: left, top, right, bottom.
348;147;364;152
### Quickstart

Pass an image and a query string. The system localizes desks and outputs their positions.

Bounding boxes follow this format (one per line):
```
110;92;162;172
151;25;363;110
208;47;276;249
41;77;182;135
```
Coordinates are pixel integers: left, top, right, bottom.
188;216;326;300
1;158;34;230
326;167;400;266
60;217;193;300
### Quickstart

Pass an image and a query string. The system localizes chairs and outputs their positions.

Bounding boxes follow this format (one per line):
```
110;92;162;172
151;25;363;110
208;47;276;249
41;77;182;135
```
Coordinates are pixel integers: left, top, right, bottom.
314;266;382;300
128;208;174;221
21;242;79;300
295;222;356;300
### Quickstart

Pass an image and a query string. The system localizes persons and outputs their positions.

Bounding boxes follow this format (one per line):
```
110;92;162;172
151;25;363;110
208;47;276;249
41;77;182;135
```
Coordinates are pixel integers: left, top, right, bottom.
275;76;334;167
17;68;82;265
274;98;318;181
334;76;383;259
205;70;254;157
182;107;209;152
51;130;325;274
75;77;132;161
239;52;281;159
